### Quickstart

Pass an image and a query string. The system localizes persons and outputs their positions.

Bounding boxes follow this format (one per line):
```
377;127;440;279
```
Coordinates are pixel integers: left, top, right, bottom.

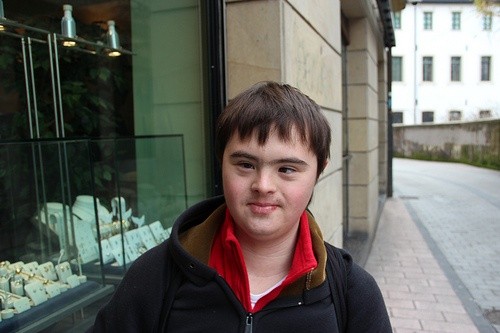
87;81;393;333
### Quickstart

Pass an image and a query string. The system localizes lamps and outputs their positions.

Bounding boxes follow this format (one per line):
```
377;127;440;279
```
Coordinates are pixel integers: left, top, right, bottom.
105;20;122;57
61;4;78;47
